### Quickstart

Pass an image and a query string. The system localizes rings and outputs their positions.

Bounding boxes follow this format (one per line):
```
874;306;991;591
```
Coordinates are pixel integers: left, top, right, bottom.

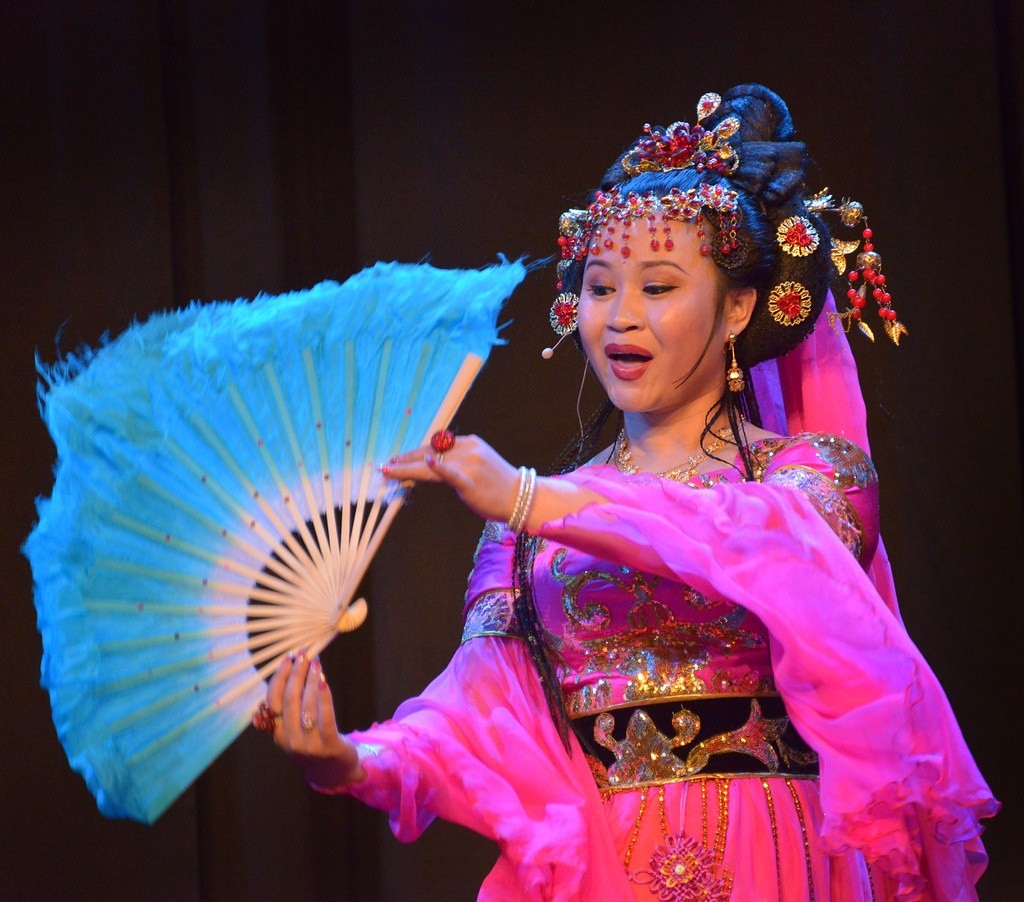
436;451;444;464
430;430;456;449
252;702;283;733
299;716;318;733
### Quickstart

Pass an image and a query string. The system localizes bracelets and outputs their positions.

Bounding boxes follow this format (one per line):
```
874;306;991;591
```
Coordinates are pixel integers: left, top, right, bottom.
504;465;538;535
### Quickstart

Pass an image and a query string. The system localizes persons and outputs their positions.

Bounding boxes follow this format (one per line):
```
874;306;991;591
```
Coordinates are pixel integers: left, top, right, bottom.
239;84;1002;902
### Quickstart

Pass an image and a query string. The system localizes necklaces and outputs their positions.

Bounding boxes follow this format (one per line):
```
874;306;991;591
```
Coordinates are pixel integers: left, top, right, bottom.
615;415;747;487
